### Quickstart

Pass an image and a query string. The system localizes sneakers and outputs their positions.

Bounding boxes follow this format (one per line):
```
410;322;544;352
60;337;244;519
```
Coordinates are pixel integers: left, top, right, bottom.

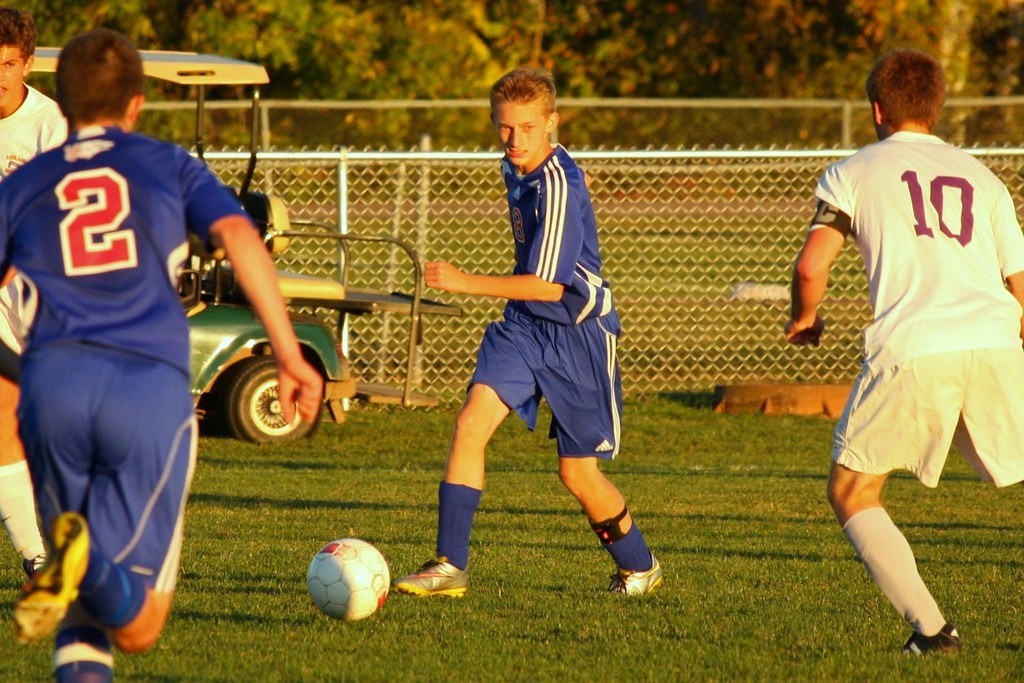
899;623;962;657
12;512;92;646
608;552;663;595
392;556;469;598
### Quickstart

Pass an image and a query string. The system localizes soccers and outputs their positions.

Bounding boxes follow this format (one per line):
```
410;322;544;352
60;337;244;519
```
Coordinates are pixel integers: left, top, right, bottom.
306;536;392;623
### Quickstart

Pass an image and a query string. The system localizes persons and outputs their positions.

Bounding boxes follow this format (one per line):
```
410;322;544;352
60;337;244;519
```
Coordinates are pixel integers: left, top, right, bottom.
0;33;322;683
397;68;663;597
0;7;69;574
783;52;1024;653
